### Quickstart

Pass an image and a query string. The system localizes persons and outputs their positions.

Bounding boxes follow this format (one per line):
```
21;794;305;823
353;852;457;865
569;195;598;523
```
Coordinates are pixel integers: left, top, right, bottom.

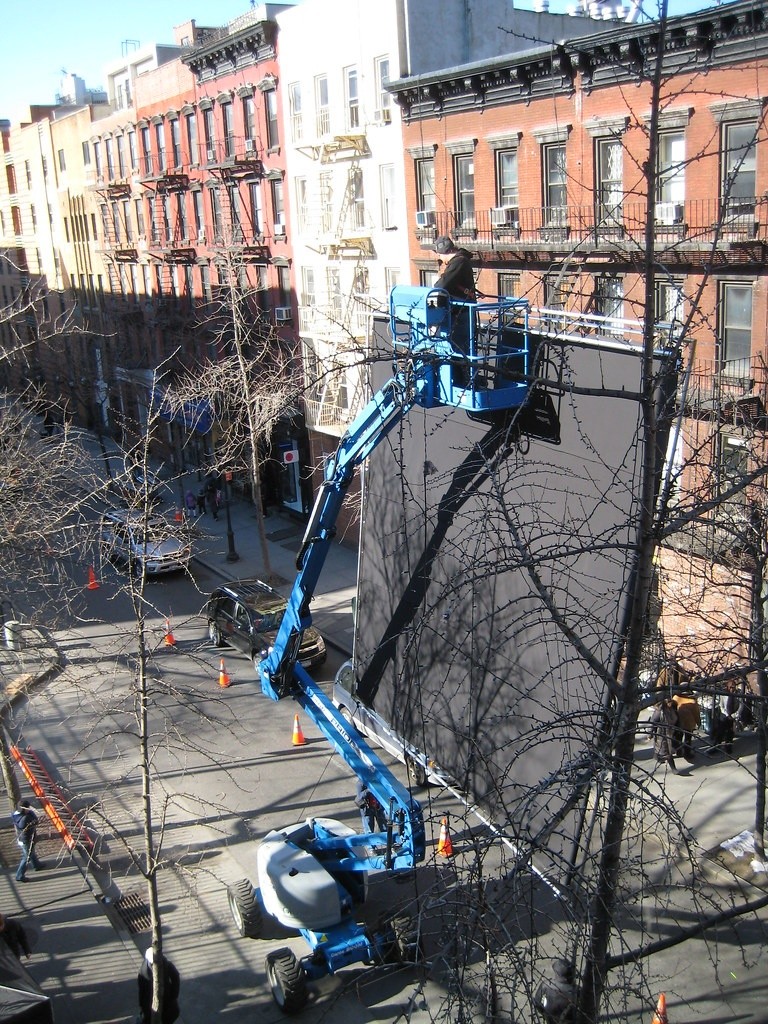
727;686;737;715
129;446;143;471
534;958;581;1024
357;779;389;833
137;947;180;1024
648;699;680;774
197;472;219;521
0;912;32;960
186;490;197;517
44;412;54;436
657;660;690;695
433;237;475;389
672;682;700;758
13;800;45;882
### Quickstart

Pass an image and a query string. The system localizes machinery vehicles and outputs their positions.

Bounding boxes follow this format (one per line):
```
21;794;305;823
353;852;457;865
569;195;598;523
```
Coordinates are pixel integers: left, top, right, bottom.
227;283;531;1016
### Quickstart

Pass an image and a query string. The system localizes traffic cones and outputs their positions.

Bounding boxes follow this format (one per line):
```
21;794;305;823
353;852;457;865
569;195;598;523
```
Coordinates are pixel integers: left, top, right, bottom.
437;818;458;859
164;621;177;646
82;565;101;589
174;505;183;522
652;994;668;1024
292;713;309;746
216;659;233;688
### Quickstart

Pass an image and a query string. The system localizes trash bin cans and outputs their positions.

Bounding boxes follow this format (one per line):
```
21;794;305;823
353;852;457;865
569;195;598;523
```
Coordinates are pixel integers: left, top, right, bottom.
39;429;48;439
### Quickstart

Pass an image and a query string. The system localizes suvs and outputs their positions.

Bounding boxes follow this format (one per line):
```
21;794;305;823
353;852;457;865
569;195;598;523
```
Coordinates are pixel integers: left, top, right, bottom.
206;578;327;679
101;509;190;581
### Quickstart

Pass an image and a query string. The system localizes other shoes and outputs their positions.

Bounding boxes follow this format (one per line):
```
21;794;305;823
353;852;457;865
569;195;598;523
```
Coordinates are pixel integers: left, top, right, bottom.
17;878;29;881
36;863;45;871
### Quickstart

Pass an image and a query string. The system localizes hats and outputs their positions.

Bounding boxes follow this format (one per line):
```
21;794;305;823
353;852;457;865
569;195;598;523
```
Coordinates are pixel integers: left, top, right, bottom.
17;800;29;812
145;948;153;962
552;960;571;977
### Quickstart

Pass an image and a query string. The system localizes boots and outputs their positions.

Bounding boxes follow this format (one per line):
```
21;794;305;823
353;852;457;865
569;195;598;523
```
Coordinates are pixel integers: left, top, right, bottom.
669;760;679;774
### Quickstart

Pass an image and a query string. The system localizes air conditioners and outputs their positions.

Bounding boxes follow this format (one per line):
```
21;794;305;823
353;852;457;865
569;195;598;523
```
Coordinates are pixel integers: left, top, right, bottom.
275;307;291;321
199;229;205;237
275;224;285;235
416;209;435;227
656;202;682;220
490;206;519;224
